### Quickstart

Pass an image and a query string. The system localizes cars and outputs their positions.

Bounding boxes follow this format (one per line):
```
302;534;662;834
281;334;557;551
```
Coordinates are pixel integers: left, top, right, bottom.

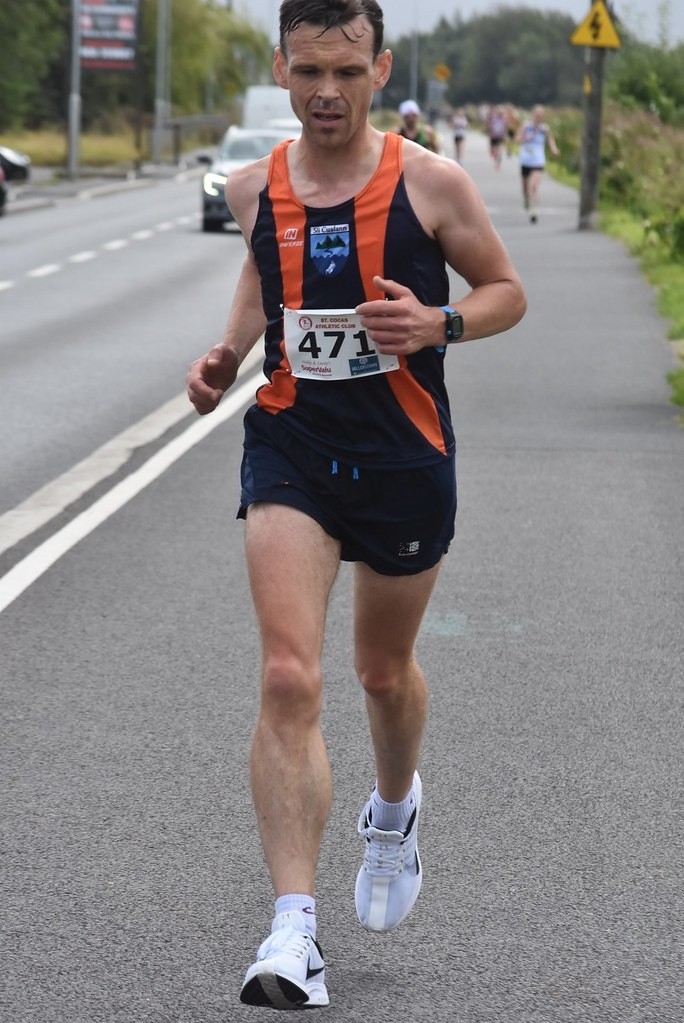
0;144;34;184
196;125;307;233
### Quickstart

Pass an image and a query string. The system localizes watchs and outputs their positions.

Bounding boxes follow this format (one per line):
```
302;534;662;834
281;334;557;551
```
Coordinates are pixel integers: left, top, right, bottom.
436;307;463;352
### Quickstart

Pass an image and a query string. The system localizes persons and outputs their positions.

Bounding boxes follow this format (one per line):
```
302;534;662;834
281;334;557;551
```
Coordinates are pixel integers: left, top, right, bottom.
396;99;439;156
186;0;526;1008
515;106;559;224
451;107;467;164
480;103;519;169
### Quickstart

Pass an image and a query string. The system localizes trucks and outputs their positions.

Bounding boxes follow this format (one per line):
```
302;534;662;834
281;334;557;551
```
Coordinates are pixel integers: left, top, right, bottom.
243;85;307;135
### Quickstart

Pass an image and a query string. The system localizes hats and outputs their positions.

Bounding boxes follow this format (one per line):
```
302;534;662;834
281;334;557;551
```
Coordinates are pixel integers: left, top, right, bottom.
400;100;418;117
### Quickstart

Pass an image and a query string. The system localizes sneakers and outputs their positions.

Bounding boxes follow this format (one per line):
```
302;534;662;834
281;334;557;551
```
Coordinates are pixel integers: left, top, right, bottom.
240;911;329;1009
355;770;422;934
